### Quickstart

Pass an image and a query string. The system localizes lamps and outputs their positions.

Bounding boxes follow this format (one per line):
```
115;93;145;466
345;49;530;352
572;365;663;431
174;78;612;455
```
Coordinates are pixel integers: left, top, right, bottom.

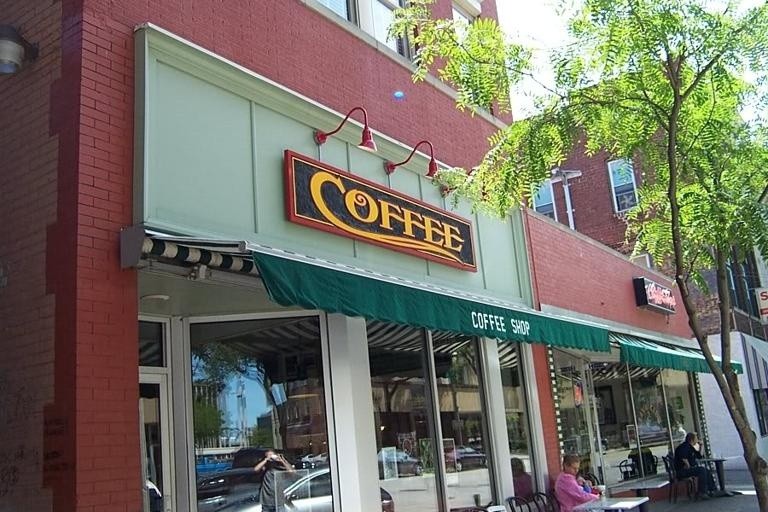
383;139;442;180
313;106;378;156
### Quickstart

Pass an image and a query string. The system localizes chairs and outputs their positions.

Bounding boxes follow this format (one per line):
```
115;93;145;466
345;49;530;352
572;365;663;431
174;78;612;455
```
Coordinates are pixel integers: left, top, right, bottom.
662;454;697;503
506;487;560;512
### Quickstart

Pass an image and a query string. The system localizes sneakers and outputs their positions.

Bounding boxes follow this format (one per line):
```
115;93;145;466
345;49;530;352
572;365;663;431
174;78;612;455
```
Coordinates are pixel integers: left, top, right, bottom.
696;490;735;500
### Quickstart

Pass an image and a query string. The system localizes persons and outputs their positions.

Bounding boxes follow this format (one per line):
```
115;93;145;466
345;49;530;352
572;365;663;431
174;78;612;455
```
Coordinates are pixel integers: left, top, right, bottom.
675;431;718;500
511;458;533;502
630;437;655;474
254;449;294;512
576;476;600;495
555;455;599;512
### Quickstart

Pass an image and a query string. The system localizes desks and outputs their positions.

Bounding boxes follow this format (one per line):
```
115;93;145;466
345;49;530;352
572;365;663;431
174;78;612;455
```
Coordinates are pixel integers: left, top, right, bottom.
628;480;670;512
573;495;650;512
697;456;742;497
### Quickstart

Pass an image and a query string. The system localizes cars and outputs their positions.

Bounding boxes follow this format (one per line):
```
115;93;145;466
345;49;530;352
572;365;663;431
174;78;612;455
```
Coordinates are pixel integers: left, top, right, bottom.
195;447;487;512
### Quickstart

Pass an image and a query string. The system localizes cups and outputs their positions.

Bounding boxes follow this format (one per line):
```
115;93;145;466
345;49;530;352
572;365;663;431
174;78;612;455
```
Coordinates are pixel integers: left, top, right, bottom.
598;484;606;501
474;494;480;506
